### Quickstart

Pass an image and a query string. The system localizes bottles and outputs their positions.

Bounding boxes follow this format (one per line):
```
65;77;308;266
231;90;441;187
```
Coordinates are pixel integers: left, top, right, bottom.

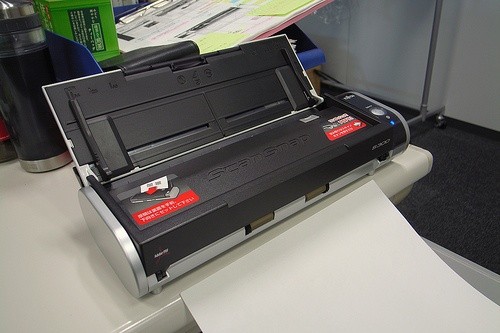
0;0;73;174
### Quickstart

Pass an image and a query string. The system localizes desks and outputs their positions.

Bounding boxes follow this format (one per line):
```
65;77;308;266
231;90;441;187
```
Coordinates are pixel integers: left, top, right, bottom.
0;144;433;333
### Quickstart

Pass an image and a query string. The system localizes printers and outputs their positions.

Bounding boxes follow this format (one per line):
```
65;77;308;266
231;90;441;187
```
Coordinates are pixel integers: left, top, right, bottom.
41;33;410;299
